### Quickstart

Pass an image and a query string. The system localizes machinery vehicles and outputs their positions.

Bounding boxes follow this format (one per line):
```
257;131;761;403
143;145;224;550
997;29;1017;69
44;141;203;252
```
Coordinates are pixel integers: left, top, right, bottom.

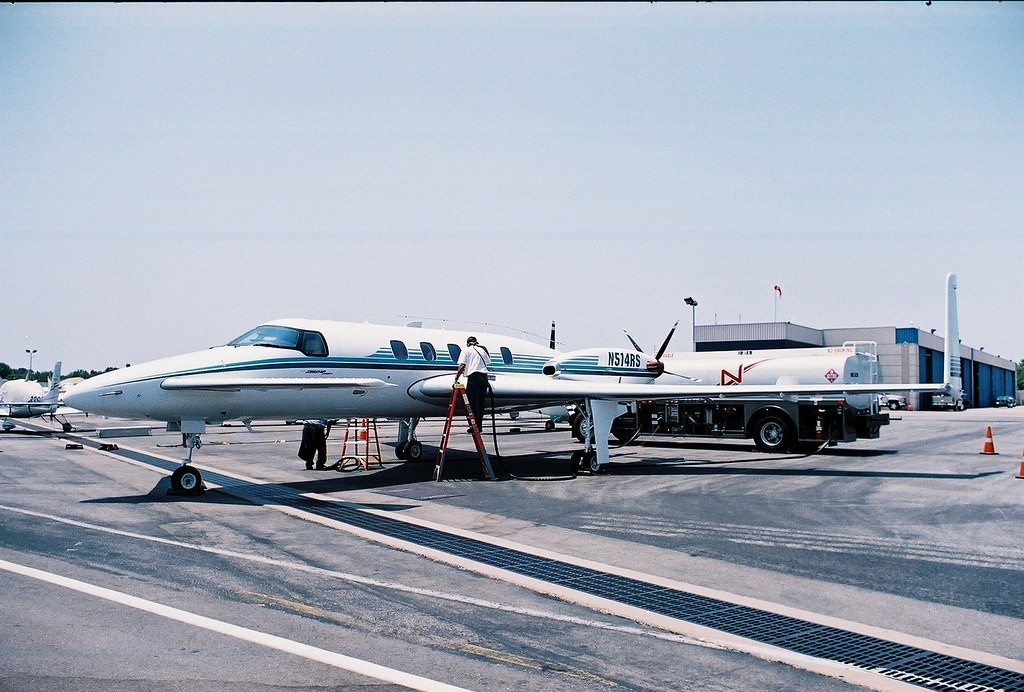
570;339;904;454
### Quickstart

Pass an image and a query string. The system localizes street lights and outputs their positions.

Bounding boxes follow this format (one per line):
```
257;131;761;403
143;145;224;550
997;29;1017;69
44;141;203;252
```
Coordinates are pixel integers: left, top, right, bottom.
26;349;38;370
683;297;699;341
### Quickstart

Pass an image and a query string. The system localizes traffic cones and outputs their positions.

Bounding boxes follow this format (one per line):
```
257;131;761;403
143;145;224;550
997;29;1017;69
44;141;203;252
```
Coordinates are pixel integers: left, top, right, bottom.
979;427;1000;455
1014;448;1024;479
908;402;912;412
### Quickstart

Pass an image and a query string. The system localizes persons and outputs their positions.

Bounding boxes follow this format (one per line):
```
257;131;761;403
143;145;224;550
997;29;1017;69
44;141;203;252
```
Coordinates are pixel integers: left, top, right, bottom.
453;337;492;433
297;419;333;471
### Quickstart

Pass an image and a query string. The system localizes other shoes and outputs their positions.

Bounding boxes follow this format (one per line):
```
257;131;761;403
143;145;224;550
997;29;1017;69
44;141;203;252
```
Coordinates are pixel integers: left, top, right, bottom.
316;463;325;469
307;462;314;470
466;425;482;433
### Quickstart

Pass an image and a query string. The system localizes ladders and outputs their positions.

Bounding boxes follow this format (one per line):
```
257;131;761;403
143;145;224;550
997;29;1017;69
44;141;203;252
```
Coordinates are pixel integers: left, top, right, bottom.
338;418;384;471
432;384;517;484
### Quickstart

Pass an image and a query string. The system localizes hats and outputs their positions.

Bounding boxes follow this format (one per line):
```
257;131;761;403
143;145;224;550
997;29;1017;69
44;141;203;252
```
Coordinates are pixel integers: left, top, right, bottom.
467;336;479;344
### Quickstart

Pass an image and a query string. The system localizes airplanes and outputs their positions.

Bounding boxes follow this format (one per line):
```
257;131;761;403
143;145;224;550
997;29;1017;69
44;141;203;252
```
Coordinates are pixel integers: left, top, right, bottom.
58;320;961;497
0;360;85;432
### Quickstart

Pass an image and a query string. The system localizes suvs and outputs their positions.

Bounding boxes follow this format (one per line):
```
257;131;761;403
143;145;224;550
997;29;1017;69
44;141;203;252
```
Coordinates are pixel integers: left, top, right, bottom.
878;391;906;411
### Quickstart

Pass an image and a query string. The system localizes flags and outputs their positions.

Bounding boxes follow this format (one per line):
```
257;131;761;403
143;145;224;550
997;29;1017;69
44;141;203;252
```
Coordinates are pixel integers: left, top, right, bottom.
774;284;782;297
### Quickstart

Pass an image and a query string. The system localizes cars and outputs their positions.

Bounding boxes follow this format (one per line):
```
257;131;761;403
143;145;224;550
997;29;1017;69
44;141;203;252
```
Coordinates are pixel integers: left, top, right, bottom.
995;397;1017;408
